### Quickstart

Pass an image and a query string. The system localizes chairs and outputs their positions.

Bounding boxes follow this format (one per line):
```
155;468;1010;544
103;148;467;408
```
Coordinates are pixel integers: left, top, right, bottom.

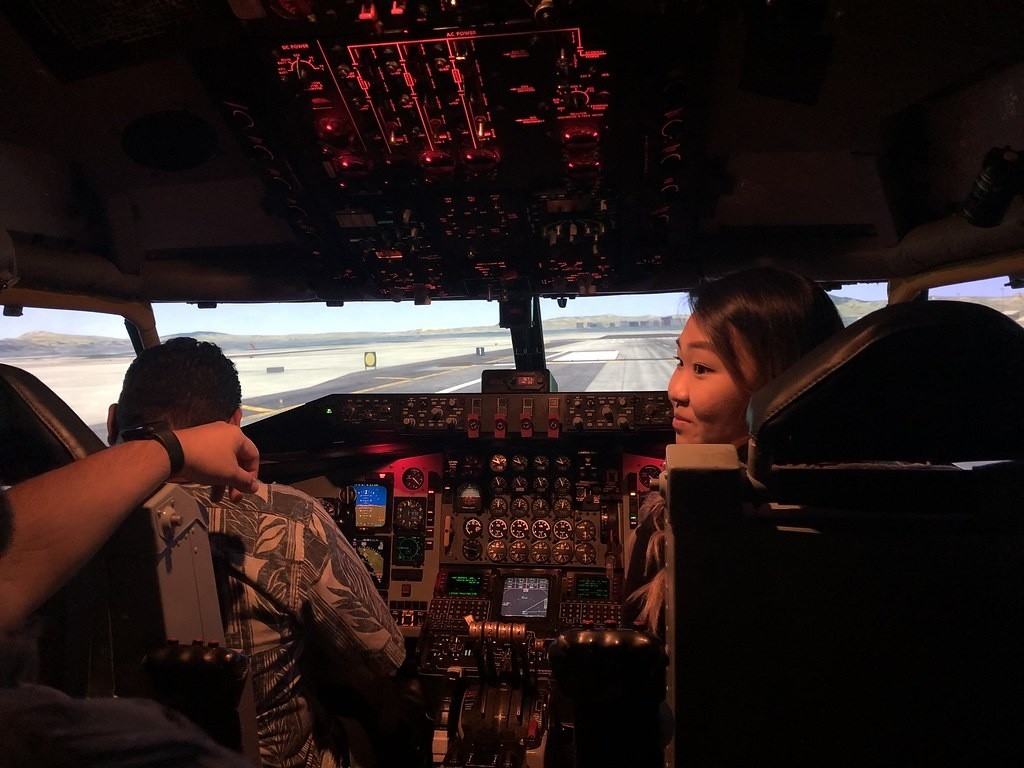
549;300;1024;768
1;364;263;767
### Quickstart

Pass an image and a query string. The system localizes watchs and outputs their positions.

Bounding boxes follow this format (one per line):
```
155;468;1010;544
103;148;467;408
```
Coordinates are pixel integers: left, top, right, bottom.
121;420;184;477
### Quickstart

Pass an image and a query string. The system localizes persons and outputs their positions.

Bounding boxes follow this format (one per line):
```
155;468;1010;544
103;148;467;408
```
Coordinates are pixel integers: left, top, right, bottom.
109;337;406;768
619;265;844;643
0;420;261;768
